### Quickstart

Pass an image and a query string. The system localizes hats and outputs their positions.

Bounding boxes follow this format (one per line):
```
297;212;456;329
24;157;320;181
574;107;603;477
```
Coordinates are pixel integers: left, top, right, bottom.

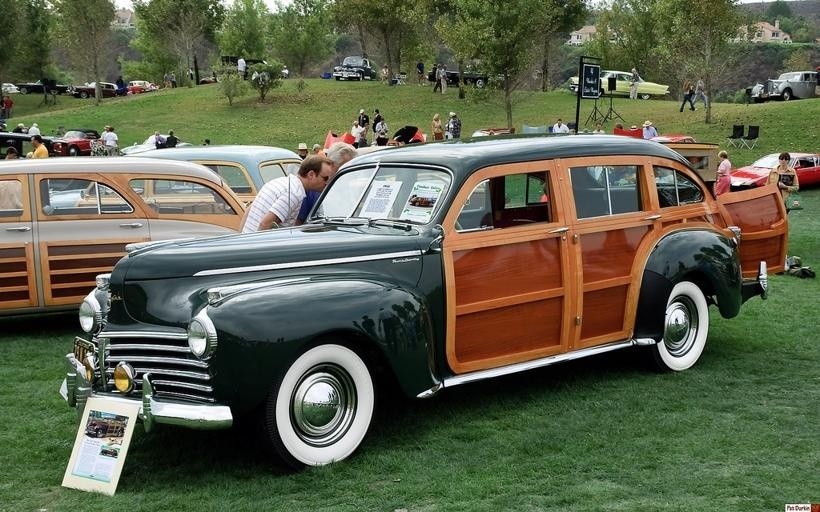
295;142;312;150
643;120;652;127
629;125;638;131
360;109;364;113
313;143;321;151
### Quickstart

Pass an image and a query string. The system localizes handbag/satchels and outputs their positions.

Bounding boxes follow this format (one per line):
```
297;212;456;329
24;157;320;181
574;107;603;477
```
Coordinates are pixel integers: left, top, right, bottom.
786;185;804;211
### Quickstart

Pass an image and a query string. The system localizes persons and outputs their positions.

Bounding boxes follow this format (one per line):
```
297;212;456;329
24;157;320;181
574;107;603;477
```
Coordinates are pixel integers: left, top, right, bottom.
679;79;698;112
714;150;731;195
692;77;707;108
0;67;218;210
765;152;799;203
380;65;388;84
237;56;246;81
239;108;659;234
416;58;449;94
629;68;639;100
281;65;289;79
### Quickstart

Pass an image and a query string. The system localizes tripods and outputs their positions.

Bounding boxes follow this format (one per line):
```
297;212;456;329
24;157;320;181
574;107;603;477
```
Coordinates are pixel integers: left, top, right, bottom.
601;90;626;125
585;99;608;125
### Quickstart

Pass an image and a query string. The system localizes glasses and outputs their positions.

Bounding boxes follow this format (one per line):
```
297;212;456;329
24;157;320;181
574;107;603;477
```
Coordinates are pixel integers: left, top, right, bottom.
779;156;789;161
317;173;329;182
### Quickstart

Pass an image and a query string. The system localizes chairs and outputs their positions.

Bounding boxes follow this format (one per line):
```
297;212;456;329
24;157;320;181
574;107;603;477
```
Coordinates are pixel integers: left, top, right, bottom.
740;125;760;150
726;124;744;148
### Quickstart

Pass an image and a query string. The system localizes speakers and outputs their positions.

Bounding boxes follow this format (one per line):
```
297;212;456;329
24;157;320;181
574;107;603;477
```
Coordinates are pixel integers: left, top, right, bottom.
599;79;601;92
608;77;617;91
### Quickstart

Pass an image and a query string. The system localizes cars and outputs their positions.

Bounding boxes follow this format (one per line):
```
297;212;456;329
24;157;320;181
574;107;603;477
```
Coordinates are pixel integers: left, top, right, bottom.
236;58;289;81
75;143;304;216
332;55;382;82
56;128;773;477
566;67;671;100
428;66;490;89
750;69;820;103
0;77;158;210
0;154;247;325
727;150;820;190
199;76;215;85
